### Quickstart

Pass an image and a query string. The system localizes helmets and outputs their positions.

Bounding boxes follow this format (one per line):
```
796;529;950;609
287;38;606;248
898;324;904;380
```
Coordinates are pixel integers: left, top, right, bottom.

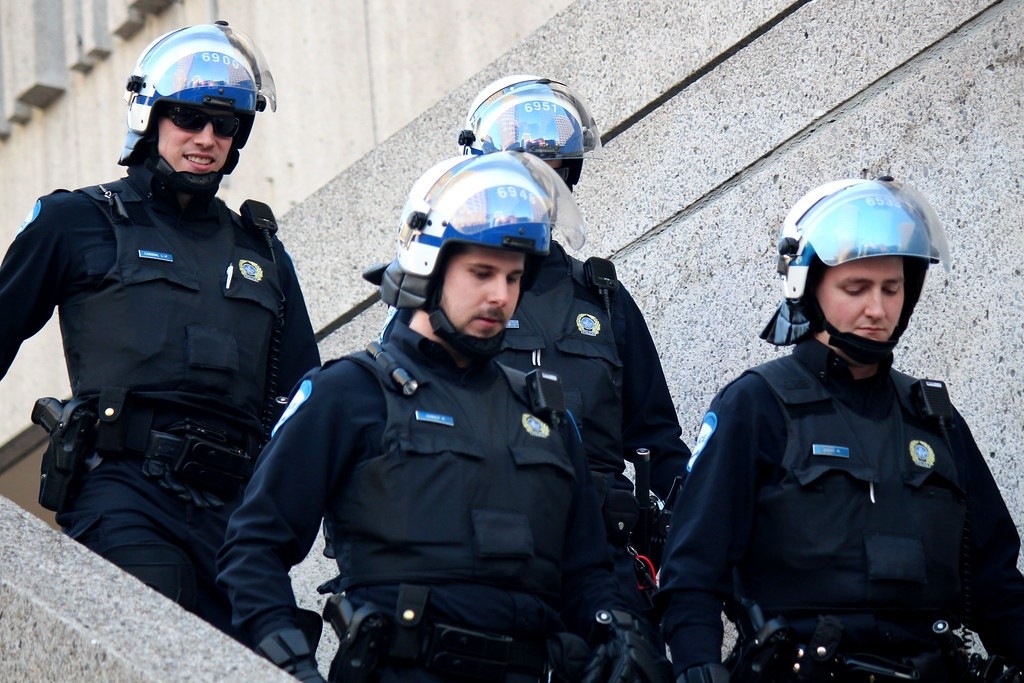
453;74;600;193
759;175;955;348
364;149;588;308
116;20;278;176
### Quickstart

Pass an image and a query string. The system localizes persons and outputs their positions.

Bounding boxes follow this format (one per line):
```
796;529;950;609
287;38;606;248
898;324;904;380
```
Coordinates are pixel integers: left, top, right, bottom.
377;74;693;549
213;152;654;683
658;179;1024;683
0;20;322;629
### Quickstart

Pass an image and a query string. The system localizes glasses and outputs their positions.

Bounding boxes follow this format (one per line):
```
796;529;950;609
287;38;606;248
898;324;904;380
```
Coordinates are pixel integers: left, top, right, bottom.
159;103;244;137
554;166;570;184
